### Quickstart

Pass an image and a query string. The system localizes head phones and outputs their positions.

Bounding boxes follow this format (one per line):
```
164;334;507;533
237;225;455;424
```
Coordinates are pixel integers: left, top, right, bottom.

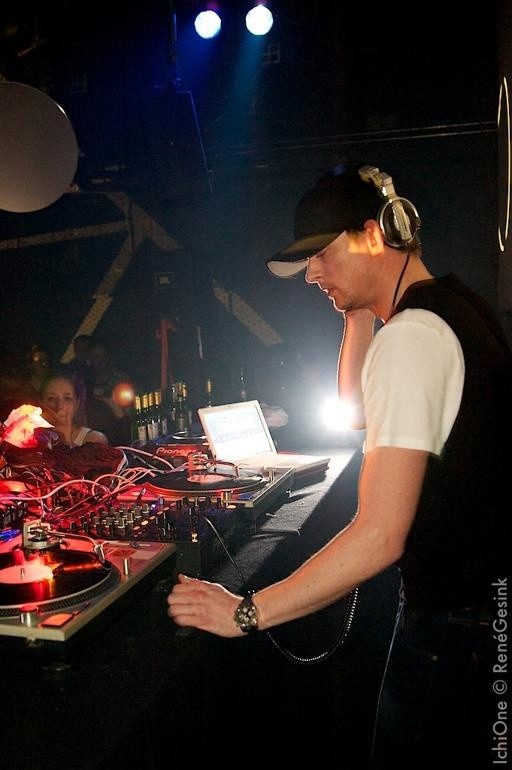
315;160;422;250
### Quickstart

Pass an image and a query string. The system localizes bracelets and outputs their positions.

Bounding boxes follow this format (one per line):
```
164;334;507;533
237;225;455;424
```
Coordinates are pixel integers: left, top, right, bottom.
232;587;261;638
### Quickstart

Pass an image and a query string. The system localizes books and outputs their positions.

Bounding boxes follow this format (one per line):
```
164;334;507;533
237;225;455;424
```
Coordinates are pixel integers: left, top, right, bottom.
1;334;132;451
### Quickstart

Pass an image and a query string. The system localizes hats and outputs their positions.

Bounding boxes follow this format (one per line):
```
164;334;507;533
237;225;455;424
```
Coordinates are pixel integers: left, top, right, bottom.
265;169;414;278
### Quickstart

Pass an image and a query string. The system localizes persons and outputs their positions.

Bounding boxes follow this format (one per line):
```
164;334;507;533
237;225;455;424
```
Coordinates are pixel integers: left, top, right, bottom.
161;164;507;765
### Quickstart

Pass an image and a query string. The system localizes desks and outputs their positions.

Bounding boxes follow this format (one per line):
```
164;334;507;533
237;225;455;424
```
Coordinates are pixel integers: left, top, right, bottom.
0;446;357;769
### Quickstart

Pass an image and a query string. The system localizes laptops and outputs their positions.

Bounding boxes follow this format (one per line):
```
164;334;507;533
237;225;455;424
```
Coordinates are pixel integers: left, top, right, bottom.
198;399;331;479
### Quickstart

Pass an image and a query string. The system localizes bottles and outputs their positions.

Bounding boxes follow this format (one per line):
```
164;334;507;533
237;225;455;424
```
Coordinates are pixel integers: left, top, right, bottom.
129;374;248;445
